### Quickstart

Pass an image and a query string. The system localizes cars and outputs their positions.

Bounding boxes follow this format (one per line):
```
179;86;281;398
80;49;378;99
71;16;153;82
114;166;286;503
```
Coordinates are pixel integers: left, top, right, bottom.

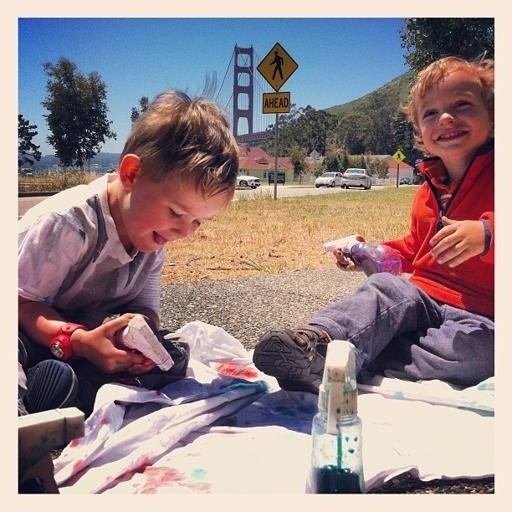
21;167;32;176
341;169;371;189
236;172;260;189
400;178;410;185
314;171;342;188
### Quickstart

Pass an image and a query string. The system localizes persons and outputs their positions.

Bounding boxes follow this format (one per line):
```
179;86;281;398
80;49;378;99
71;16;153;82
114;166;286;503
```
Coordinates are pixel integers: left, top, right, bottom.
19;88;242;417
250;54;495;398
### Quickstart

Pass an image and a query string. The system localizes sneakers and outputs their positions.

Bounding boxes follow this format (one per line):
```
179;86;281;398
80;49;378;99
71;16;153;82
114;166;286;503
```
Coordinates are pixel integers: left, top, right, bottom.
252;324;329;395
23;359;79;414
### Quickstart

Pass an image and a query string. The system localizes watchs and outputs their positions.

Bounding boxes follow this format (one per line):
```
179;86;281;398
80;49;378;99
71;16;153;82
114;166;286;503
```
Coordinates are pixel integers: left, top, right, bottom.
47;322;91;362
